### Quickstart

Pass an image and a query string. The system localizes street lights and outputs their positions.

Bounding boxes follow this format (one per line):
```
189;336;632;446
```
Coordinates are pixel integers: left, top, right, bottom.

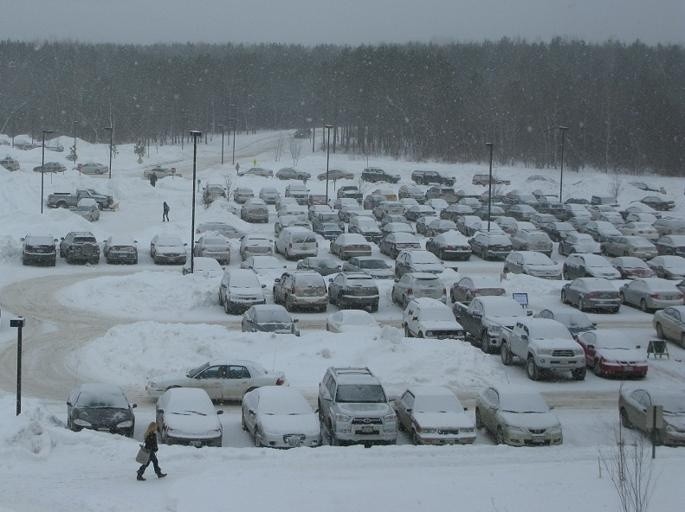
324;124;333;205
485;142;494;233
558;125;569;203
189;130;201;273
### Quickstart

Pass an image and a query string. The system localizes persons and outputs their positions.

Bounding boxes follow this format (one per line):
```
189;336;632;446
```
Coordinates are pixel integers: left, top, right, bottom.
135;420;167;481
150;171;157;189
161;201;171;223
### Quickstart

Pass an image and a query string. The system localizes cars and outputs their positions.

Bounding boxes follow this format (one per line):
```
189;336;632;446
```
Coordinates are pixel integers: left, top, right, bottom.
259;186;280;204
76;161;110;175
477;384;564;448
238;167;274;177
575;330;648;379
195;220;241;239
317;170;355;181
240;233;274;262
618;278;685;313
152;385;229;451
63;381;136;441
324;309;384;341
560;277;621;313
240;304;301;337
628;182;667;195
35;161;70;180
394;380;484;448
144;162;183;180
525;174;558;184
150;229;192;270
194;229;235;268
449;275;507;303
233;187;255;204
291;123;322;147
275;167;311;181
104;227;142;268
617;384;685;449
240;382;322;451
652;305;684;350
165;355;292;385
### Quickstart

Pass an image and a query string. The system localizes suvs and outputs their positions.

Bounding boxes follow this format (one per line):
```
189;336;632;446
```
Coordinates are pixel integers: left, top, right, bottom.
21;231;57;267
59;228;101;268
402;290;466;343
202;183;227;204
411;169;457;187
391;272;447;310
315;363;402;448
218;268;267;314
272;269;328;313
472;174;511;186
327;271;379;312
273;188;685;279
241;198;269;224
361;167;402;184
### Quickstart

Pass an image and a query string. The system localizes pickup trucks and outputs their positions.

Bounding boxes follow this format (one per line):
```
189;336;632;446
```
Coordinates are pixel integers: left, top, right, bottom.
451;295;534;354
491;313;587;380
44;189;116;211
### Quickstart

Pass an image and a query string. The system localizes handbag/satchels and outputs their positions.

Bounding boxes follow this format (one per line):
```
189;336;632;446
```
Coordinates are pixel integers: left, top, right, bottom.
136;448;151;465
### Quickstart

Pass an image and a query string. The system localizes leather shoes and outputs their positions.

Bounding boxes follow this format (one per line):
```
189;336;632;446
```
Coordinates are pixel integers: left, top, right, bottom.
137;476;146;480
158;473;167;478
